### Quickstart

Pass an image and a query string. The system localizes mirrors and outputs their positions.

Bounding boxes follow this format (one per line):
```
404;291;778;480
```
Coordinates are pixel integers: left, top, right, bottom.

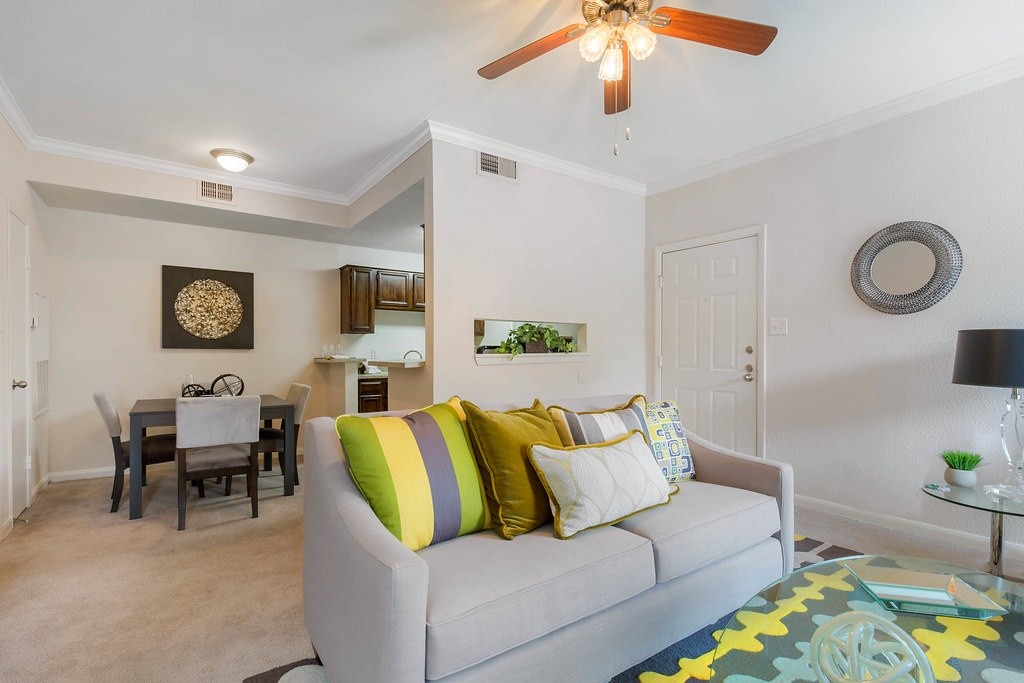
849;222;962;313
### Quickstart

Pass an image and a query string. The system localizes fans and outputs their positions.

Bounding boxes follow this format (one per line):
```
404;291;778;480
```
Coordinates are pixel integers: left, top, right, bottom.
477;1;778;115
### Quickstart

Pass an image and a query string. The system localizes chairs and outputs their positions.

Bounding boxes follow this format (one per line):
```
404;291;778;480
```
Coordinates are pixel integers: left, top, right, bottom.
173;394;266;531
94;390;180;514
257;382;318;486
183;380;239;486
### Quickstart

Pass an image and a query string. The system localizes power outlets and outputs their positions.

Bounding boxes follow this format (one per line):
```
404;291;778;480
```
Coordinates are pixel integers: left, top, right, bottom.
371;348;379;359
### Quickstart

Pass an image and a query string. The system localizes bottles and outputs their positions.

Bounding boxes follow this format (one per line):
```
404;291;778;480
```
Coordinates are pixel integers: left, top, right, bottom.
474;344;477;354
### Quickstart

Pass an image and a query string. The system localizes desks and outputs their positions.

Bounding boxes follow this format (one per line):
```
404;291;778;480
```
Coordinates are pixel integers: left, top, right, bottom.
127;393;298;520
924;486;1022;575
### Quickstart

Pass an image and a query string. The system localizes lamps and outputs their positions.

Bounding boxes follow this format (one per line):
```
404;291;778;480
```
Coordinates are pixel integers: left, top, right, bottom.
574;2;670;84
208;147;255;174
951;329;1024;500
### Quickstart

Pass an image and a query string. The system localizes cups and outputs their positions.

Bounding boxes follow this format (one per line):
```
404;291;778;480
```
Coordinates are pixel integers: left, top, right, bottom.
371;348;377;359
321;344;341;356
184;374;193;395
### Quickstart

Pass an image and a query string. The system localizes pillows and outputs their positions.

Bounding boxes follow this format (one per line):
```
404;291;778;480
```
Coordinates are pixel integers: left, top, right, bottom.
456;399;563;540
336;394;496;553
521;430;674;539
552;396;679;497
642;401;697;483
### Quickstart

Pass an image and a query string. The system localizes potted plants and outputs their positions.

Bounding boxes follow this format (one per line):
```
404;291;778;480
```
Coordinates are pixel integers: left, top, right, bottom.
940;449;985;488
496;326;572;359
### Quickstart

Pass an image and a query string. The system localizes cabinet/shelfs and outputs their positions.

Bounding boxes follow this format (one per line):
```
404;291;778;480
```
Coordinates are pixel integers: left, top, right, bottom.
357;379;387;412
338;264;375;335
379;268;424;312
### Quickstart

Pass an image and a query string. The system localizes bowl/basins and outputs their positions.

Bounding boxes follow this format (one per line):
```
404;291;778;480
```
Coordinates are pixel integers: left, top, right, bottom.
377;366;388;372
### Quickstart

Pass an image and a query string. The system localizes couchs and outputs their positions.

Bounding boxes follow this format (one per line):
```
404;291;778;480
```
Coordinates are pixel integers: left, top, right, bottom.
299;391;801;682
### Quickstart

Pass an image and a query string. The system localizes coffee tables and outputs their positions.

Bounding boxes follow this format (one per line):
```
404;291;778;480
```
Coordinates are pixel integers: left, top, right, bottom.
710;554;1023;683
